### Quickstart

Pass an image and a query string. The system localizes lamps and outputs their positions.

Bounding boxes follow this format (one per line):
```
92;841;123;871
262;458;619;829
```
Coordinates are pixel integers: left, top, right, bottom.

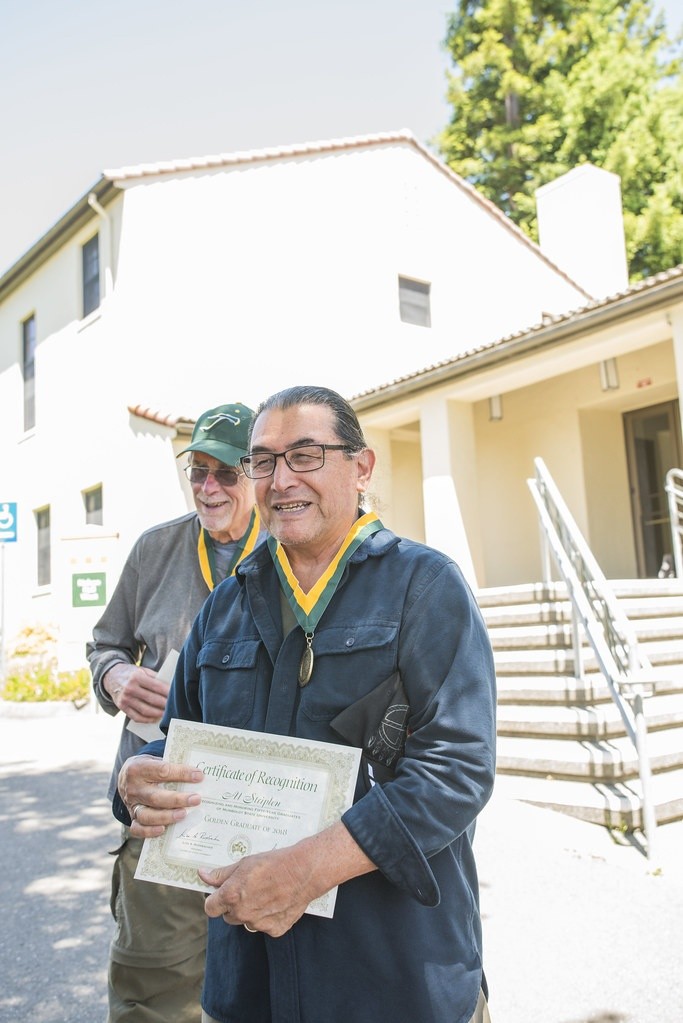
598;358;619;392
487;395;502;423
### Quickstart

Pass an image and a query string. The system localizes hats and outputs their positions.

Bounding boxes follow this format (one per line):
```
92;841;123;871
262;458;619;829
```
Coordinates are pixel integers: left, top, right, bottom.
176;402;258;467
330;669;410;794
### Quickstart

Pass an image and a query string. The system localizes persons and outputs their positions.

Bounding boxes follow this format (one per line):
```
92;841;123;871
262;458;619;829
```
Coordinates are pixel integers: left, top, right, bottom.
112;385;492;1023
86;401;271;1023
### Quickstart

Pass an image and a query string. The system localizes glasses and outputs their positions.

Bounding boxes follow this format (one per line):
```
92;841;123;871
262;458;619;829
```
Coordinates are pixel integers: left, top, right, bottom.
239;444;349;480
183;463;246;486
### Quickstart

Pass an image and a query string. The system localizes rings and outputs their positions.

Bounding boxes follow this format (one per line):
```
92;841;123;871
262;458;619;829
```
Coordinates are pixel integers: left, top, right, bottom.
244;924;258;933
133;805;140;818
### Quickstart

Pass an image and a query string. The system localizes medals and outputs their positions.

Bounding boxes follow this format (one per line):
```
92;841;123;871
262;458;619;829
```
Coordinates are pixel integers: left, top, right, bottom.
298;643;314;687
